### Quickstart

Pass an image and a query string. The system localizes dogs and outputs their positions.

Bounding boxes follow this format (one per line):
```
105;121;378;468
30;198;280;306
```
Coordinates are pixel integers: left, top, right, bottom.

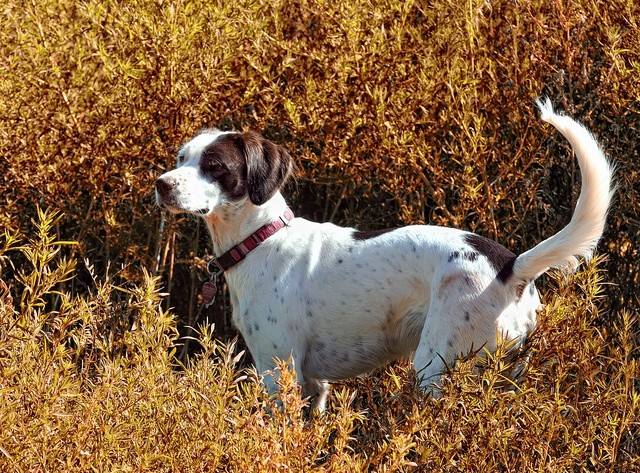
152;96;619;418
